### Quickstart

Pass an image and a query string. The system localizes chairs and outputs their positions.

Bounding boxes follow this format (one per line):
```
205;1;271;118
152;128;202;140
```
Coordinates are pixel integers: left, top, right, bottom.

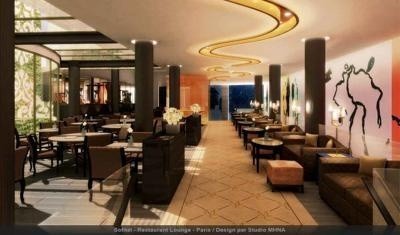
12;104;201;207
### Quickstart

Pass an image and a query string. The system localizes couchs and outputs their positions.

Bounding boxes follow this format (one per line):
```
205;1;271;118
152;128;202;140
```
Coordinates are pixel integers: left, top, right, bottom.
228;98;400;234
